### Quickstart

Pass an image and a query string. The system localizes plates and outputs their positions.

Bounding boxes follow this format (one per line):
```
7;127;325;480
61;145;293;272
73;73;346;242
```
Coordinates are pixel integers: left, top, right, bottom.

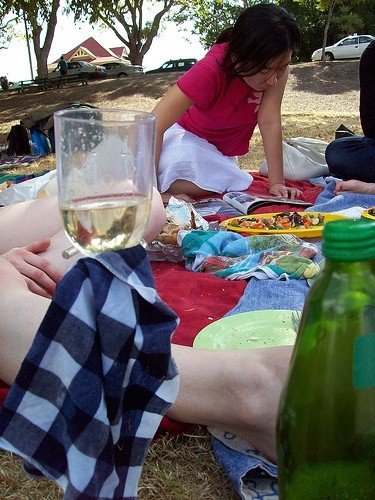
192;309;302;350
361;207;375;220
220;212;346;238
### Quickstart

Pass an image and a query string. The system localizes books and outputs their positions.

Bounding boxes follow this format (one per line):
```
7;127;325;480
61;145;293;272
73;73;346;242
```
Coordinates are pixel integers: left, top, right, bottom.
182;191;313;221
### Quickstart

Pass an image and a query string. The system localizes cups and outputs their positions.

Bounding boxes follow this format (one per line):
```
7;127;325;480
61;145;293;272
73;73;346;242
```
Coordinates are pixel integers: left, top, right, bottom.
53;108;158;258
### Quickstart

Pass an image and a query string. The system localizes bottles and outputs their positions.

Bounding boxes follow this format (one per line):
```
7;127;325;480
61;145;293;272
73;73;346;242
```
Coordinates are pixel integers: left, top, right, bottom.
277;216;375;500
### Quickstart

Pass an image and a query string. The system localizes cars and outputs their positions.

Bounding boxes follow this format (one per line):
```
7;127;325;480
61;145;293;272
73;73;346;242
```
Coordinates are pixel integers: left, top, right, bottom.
99;61;143;78
35;61;107;86
144;57;198;78
311;32;375;62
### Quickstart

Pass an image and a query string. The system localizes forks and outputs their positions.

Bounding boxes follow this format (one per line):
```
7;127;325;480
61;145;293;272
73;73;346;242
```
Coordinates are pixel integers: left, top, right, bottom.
291;309;303;332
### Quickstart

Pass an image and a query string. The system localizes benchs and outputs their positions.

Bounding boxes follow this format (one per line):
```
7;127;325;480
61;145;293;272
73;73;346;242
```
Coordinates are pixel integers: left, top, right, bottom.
15;78;50;94
53;73;88;89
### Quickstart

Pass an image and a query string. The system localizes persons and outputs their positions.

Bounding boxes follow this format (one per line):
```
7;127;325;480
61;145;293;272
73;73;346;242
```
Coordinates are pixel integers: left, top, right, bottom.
326;36;375;198
0;187;294;465
57;56;68;86
150;3;310;198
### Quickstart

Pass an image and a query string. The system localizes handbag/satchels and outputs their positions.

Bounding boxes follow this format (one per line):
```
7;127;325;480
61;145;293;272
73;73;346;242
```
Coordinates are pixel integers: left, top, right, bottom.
6;125;55;158
